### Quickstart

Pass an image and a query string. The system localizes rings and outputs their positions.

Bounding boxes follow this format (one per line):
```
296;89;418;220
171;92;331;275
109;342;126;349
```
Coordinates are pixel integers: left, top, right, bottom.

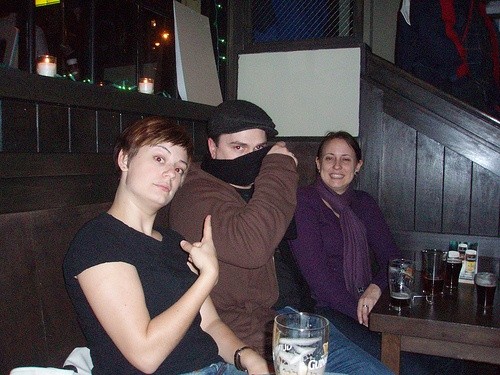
363;305;367;307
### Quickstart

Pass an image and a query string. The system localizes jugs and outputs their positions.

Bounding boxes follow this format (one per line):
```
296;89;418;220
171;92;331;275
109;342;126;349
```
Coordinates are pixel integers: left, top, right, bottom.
414;248;447;296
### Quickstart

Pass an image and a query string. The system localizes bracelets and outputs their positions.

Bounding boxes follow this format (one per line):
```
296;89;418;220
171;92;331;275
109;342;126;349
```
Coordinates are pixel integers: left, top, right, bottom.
233;346;258;372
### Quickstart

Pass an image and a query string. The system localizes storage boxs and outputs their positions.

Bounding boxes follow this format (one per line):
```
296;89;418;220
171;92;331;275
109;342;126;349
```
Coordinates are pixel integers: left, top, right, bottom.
449;240;479;285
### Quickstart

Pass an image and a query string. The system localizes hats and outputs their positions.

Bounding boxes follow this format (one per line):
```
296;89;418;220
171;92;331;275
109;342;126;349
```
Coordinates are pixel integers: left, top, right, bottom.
207;99;279;143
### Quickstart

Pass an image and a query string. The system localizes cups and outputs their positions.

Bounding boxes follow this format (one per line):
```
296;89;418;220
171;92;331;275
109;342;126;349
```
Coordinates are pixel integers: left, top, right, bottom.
389;258;415;300
443;255;463;294
139;77;154;94
475;272;497;309
36;54;58;78
272;312;330;375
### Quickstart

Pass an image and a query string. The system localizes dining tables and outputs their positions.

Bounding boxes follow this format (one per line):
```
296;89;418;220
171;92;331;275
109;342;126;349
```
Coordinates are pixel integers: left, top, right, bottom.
370;256;500;375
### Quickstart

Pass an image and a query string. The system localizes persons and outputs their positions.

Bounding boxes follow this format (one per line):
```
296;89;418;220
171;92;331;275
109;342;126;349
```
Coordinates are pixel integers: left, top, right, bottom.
61;115;277;375
169;100;396;375
283;130;470;375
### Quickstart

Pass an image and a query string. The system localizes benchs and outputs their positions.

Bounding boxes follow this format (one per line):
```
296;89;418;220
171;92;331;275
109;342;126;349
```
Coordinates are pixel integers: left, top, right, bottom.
0;203;169;374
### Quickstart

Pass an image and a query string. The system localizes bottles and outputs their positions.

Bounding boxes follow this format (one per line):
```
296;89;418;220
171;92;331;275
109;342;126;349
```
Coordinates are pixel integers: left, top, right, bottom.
66;58;80;79
448;239;476;272
